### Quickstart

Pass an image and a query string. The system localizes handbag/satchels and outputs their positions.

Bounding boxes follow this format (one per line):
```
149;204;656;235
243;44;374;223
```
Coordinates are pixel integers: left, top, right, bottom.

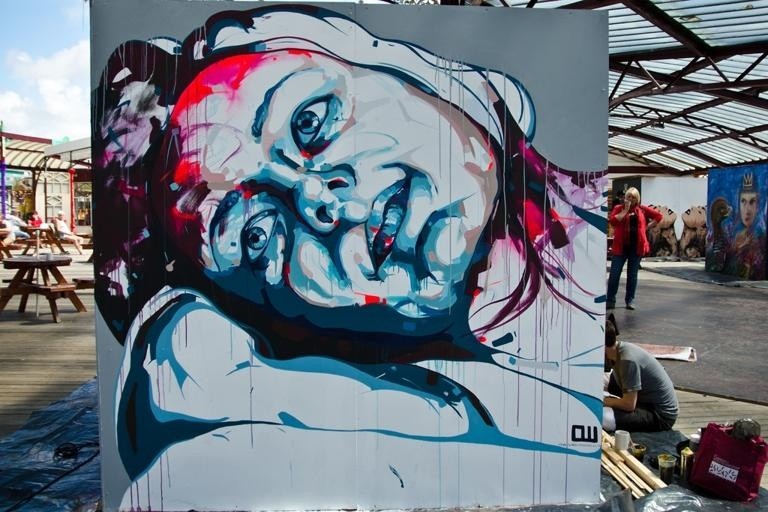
687;422;768;503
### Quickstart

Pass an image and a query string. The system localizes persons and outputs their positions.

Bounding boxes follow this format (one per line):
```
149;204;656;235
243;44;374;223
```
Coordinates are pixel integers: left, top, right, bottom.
607;185;663;311
731;188;761;279
674;205;707;261
0;206;92;264
89;6;603;395
601;320;680;436
643;204;678;256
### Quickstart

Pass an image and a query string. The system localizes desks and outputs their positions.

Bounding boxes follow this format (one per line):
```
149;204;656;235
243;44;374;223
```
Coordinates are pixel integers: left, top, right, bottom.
0;257;87;324
21;225;51;232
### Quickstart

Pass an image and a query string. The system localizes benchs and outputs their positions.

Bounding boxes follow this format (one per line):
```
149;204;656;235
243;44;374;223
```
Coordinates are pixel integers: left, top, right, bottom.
0;277;94;324
0;239;80;251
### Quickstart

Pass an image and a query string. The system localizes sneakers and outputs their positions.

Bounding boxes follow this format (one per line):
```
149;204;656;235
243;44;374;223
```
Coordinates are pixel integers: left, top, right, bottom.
606;302;615;309
626;305;635;310
